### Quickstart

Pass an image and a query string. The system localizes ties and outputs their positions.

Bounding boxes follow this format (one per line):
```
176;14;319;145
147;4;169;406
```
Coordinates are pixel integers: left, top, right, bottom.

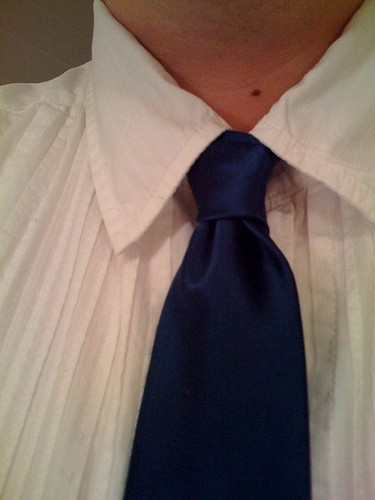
118;130;312;499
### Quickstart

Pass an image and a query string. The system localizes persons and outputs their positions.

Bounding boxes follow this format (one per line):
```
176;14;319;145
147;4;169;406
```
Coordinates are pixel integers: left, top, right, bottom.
0;0;375;499
